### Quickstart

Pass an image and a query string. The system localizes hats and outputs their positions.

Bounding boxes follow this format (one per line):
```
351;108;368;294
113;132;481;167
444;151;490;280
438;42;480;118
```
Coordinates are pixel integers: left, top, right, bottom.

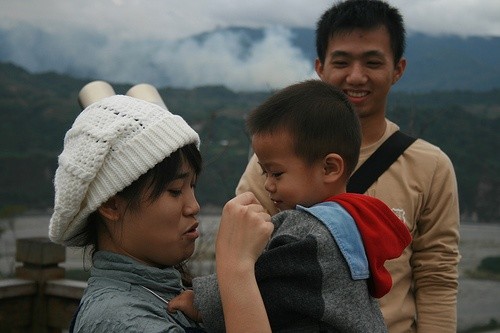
48;93;202;247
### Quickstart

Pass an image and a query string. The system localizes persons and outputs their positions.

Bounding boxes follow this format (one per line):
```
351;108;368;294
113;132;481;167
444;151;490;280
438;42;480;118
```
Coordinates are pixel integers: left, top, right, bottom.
49;95;273;333
236;0;460;332
168;80;412;333
